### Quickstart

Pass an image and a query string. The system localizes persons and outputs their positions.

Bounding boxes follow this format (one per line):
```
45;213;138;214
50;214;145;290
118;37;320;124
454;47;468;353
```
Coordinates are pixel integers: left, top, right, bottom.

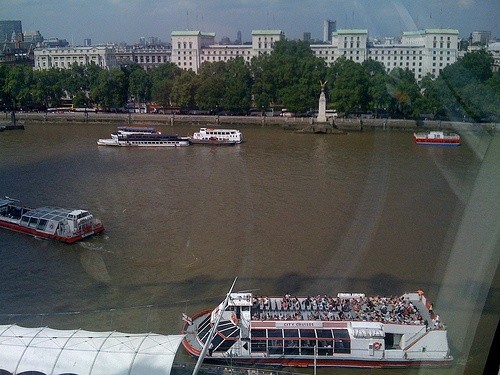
240;288;448;329
207;342;216;357
372;341;383;351
275;338;346;350
401;352;409;360
381;349;386;360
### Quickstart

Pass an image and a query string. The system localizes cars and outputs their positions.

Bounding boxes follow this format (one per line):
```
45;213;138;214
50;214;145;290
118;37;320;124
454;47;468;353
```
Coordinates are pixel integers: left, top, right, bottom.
45;99;436;121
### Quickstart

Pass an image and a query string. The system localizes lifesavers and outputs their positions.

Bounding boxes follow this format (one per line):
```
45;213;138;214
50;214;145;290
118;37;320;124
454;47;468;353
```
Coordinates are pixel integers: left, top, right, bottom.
373;342;381;350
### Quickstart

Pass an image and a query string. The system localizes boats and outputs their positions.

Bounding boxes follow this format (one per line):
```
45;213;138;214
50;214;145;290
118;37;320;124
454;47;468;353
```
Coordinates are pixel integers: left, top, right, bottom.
180;291;455;369
0;194;105;244
413;130;462;147
0;323;313;375
96;126;244;147
